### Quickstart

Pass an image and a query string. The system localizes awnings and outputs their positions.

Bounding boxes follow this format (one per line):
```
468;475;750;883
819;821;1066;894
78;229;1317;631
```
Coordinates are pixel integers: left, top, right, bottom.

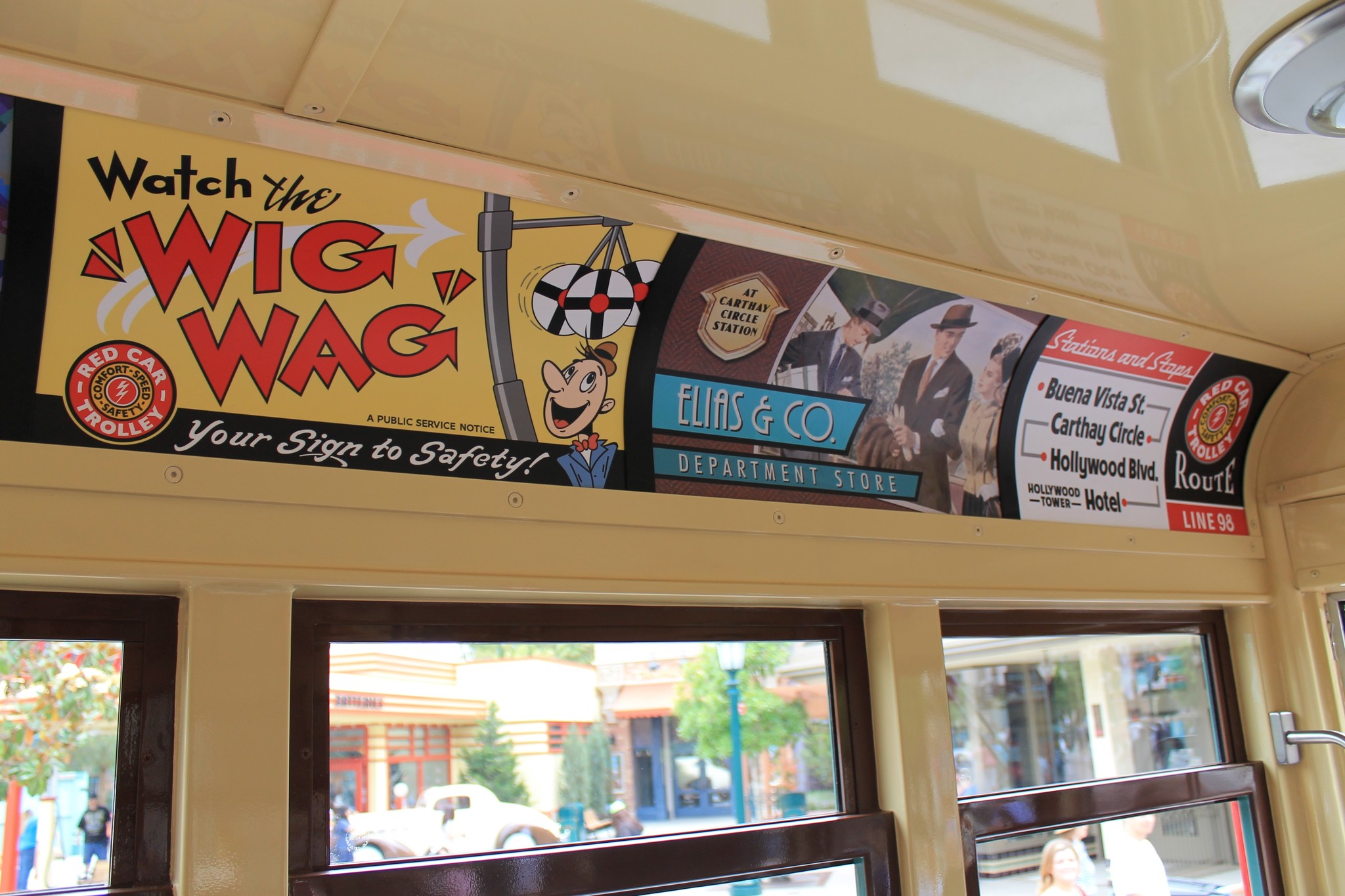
611;683;679;719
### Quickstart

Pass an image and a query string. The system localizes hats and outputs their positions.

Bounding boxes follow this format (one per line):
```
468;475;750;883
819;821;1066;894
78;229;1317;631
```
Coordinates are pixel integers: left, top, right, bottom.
609;800;626;814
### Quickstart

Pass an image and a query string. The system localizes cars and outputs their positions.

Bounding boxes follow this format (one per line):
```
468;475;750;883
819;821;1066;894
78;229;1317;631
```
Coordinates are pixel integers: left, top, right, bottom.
350;784;563;865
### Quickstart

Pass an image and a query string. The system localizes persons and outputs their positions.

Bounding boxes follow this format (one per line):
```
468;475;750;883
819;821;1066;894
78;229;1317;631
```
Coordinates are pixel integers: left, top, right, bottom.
73;792;112;882
14;809;38;892
880;306;973;513
1039;824;1106;896
775;298;892;403
608;800;644;840
955;335;1021;519
1108;812;1176;896
1037;839;1099;896
330;805;356;863
